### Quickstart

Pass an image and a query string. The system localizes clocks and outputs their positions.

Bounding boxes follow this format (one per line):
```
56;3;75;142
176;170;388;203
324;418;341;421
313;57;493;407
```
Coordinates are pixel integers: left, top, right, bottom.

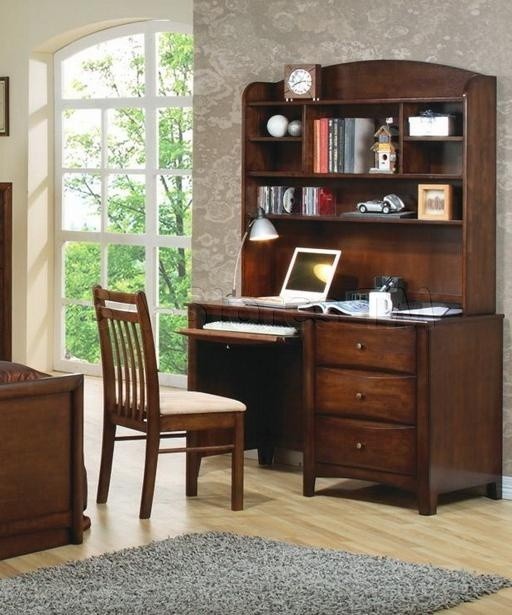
284;64;322;102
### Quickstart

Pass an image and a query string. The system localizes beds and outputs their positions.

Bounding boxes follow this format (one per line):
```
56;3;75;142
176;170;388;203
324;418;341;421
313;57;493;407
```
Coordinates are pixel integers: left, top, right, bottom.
0;182;91;560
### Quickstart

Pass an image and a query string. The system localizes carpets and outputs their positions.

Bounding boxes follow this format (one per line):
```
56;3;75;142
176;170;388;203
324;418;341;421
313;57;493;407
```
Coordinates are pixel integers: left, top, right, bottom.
0;531;512;615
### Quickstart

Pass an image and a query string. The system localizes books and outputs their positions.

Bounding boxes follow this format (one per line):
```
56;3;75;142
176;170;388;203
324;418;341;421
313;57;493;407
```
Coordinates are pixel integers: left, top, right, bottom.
313;117;379;172
297;298;369;314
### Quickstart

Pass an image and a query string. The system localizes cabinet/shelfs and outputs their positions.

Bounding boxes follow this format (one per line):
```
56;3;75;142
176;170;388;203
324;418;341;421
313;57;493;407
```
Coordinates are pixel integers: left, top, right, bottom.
174;60;505;516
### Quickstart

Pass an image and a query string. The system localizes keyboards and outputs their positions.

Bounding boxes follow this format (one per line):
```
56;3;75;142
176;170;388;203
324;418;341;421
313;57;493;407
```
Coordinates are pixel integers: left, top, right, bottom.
203;320;299;336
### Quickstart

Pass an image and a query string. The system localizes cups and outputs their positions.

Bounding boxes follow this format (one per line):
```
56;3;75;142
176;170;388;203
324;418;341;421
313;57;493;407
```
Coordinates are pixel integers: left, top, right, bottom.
369;291;393;318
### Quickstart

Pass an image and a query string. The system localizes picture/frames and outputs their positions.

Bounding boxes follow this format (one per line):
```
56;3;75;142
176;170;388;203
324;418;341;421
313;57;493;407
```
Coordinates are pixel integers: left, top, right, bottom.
418;184;454;221
0;77;9;136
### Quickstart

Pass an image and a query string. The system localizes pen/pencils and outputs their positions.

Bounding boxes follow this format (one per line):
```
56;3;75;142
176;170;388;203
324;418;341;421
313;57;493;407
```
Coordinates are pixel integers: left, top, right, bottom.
377;277;395;291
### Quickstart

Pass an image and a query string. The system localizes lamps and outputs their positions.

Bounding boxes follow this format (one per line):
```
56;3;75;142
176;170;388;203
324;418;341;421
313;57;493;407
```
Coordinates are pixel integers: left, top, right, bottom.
223;206;279;303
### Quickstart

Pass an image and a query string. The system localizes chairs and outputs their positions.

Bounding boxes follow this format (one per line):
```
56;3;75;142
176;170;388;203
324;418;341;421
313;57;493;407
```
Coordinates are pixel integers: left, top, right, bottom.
94;286;246;519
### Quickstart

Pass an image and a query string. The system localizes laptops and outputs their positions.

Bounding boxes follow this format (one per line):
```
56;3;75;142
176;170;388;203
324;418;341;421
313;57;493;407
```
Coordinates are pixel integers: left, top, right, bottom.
242;247;342;309
